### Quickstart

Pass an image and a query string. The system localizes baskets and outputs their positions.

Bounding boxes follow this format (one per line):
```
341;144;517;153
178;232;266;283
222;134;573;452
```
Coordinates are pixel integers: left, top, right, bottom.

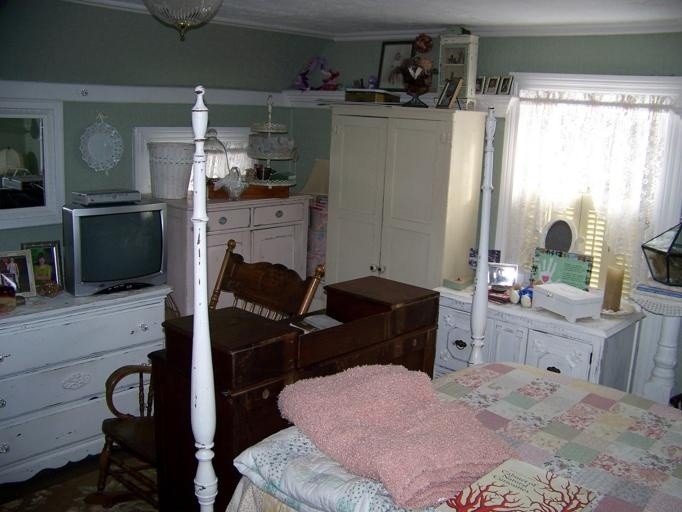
146;140;197;202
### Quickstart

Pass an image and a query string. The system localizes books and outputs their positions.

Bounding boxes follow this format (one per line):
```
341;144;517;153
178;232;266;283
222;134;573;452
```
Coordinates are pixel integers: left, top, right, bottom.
290;321;319;334
304;313;345;332
434;457;595;512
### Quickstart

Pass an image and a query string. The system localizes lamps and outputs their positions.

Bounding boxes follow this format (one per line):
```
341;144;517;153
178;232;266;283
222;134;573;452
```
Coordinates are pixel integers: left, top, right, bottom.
145;0;222;39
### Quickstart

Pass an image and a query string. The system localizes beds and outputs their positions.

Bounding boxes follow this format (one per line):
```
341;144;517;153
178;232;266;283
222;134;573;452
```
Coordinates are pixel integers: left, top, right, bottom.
223;360;682;511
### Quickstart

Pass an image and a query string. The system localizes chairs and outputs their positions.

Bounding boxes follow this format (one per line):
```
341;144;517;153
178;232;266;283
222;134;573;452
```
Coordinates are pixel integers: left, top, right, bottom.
95;242;325;511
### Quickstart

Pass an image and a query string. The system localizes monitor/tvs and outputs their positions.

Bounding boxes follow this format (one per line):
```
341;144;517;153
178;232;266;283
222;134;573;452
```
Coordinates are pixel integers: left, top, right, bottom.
63;199;168;298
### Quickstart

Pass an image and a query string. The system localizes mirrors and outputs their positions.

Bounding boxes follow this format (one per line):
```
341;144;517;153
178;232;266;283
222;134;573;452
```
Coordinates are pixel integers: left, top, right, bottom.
540;216;576;253
79;122;125;172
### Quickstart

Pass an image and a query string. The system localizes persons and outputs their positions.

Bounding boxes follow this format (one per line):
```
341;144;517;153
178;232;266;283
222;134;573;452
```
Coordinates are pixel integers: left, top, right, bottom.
32;251;52;287
5;257;22;289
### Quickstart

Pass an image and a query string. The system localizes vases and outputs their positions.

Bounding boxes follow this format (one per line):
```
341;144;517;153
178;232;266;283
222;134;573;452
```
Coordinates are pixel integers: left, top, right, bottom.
402;82;430;108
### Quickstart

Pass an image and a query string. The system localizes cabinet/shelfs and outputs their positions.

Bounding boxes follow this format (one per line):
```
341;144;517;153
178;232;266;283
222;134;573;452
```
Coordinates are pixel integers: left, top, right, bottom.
147;276;440;510
141;192;312;316
0;282;175;486
433;285;647;393
438;33;479;109
326;102;488;291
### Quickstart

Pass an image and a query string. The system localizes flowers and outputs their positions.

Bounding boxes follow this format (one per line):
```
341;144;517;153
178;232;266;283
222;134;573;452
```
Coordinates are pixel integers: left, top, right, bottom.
392;53;439;96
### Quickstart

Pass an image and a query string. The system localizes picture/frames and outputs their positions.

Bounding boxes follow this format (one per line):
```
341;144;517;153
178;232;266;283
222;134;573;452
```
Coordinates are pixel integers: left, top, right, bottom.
530;247;594;291
0;248;36;297
487;262;519;288
435;77;464;110
0;97;65;229
476;75;513;95
377;39;415;92
21;241;63;290
